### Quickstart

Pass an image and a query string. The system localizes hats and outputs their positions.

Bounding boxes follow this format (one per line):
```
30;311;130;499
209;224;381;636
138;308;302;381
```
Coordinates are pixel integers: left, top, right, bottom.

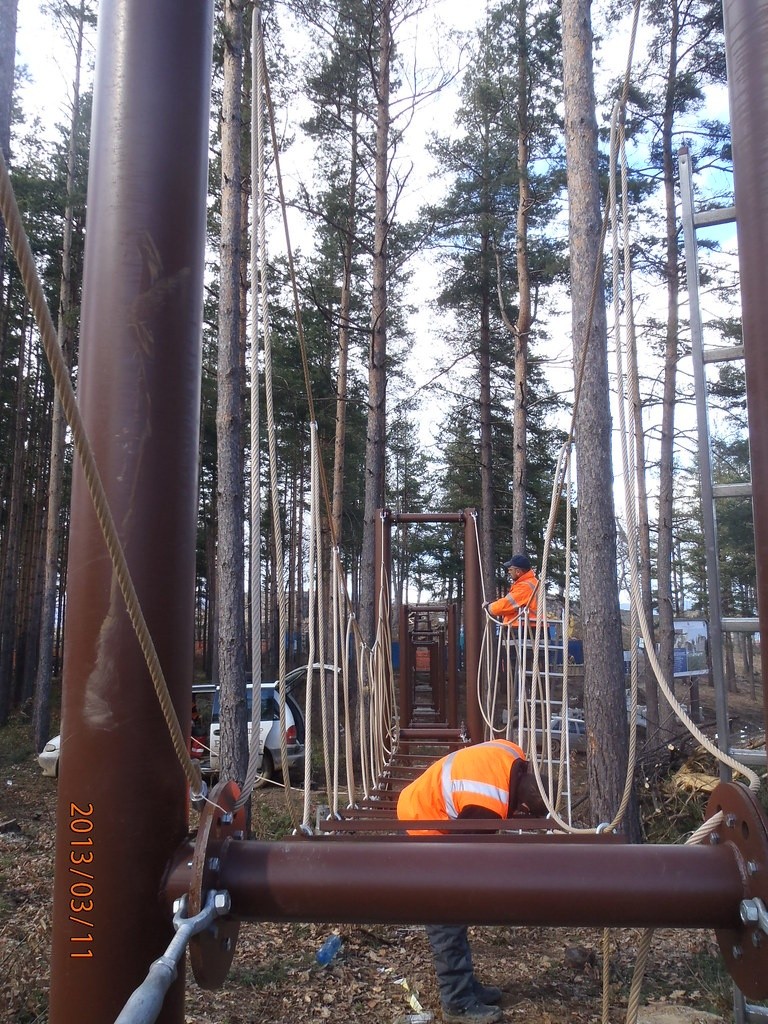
504;554;531;569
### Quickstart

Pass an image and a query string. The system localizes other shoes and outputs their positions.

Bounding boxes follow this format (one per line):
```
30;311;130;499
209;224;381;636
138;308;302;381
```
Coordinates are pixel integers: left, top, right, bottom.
469;984;502;1003
442;1001;502;1024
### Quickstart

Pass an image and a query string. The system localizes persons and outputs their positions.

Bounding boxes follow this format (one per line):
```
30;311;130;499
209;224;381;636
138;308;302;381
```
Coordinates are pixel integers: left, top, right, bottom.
397;739;558;1024
486;555;551;729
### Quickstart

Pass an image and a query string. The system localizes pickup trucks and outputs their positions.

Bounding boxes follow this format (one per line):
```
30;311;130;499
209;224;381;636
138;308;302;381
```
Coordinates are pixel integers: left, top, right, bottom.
516;717;588;755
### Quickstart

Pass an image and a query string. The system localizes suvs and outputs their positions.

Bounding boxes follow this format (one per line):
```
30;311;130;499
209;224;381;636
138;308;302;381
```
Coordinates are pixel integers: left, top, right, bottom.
35;663;343;792
